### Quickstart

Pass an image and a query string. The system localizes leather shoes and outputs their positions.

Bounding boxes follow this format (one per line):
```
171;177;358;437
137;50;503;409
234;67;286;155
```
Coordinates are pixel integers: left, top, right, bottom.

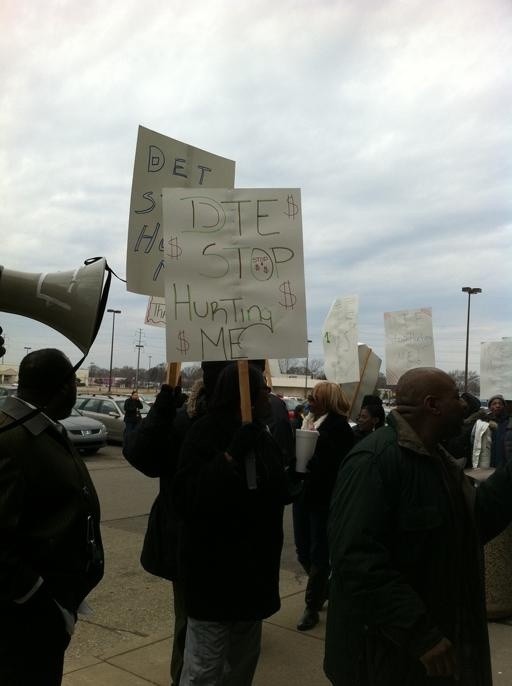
297;613;319;631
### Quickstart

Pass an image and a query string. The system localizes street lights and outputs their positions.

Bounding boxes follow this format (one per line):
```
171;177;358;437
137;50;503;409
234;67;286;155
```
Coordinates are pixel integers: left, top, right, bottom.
462;286;482;392
106;309;121;391
135;344;143;392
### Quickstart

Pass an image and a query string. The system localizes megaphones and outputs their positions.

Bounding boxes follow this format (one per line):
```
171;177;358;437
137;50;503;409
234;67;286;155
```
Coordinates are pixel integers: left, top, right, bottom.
0;255;107;357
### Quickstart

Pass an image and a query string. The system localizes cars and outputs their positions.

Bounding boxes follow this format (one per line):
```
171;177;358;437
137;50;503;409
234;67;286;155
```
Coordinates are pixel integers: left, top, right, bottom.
0;384;108;456
72;393;152;445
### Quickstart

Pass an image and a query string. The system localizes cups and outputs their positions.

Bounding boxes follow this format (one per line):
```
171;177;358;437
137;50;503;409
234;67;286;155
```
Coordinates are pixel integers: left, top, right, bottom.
295;428;320;474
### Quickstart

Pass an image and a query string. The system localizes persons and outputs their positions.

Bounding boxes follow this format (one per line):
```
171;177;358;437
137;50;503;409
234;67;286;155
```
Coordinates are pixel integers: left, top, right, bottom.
120;360;512;686
0;348;104;686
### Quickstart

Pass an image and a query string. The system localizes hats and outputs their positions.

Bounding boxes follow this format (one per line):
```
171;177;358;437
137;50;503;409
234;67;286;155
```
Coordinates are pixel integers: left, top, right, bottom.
488;395;505;408
